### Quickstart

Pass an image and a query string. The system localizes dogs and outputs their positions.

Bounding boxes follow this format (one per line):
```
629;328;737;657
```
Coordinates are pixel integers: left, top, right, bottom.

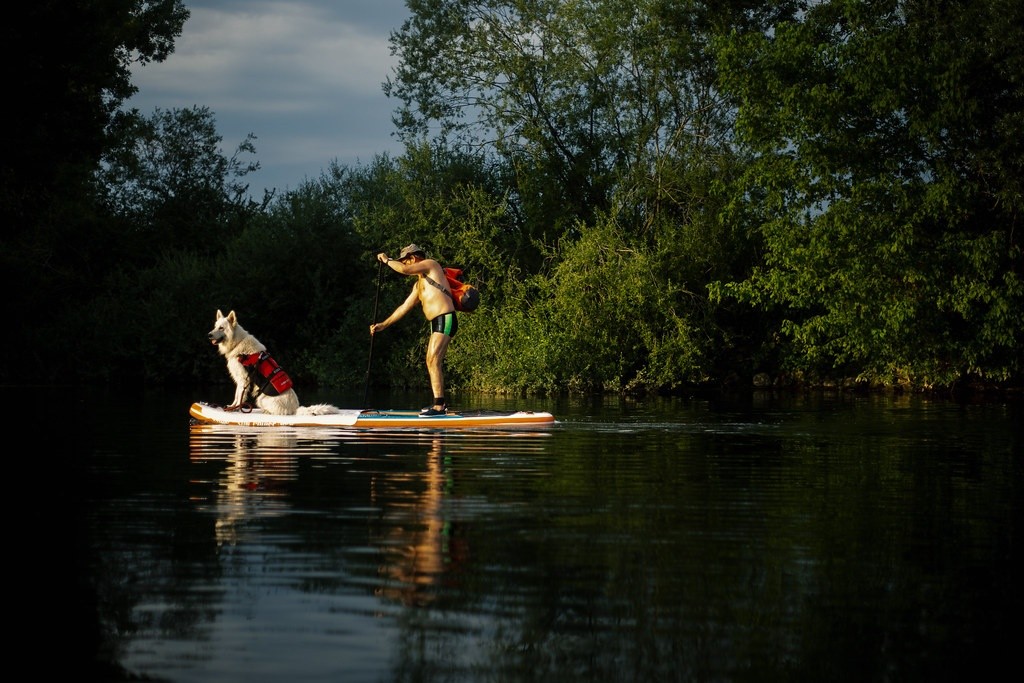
206;308;342;418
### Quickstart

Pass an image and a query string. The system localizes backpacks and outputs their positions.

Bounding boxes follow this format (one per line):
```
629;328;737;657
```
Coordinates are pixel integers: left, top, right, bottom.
422;263;480;313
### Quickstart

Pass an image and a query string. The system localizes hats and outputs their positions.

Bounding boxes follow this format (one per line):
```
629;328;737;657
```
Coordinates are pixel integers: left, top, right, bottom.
396;243;425;261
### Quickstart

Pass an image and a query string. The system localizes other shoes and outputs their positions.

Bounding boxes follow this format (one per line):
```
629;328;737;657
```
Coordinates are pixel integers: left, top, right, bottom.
418;408;446;417
421;406;448;413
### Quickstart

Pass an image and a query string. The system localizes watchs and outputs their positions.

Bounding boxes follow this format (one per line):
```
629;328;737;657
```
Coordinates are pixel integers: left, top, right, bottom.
386;258;393;265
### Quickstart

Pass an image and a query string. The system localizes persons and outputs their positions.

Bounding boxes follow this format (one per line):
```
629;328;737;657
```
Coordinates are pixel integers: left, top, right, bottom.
370;244;458;417
